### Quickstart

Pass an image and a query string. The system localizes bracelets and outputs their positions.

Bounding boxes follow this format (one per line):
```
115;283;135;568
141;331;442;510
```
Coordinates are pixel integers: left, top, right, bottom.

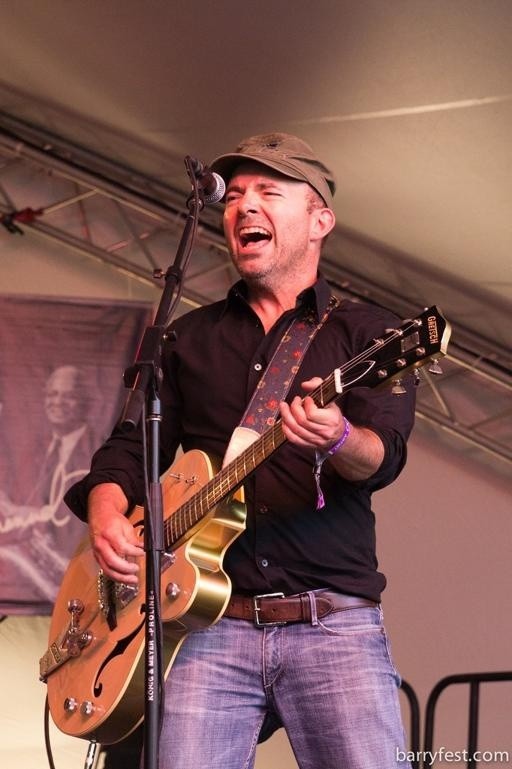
311;416;353;508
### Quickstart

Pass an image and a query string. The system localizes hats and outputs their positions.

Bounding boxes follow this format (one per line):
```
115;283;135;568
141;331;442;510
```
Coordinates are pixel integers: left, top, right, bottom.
201;132;338;215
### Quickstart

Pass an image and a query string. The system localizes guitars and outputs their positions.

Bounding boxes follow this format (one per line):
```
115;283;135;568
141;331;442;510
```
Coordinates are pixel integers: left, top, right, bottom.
39;305;451;746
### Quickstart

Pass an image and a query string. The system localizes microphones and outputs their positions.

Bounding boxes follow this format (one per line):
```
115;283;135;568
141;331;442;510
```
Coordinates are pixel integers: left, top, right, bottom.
184;150;230;208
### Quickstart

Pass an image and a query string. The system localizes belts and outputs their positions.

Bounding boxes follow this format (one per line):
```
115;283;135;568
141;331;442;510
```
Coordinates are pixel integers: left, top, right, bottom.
221;582;378;626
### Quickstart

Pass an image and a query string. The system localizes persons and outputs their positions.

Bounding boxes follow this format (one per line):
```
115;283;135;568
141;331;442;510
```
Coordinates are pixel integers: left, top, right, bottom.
60;130;419;768
1;360;105;602
